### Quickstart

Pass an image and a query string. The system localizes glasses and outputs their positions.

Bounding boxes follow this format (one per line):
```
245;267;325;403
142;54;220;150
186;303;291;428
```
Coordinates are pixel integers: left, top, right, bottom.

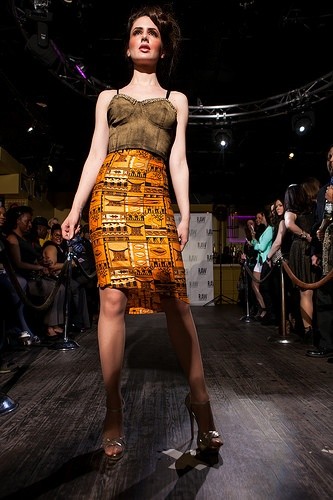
54;233;62;237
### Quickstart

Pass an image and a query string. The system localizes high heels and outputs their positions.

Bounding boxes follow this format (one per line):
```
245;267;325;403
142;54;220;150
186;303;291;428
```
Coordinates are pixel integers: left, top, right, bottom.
185;392;224;452
300;326;313;346
100;398;126;461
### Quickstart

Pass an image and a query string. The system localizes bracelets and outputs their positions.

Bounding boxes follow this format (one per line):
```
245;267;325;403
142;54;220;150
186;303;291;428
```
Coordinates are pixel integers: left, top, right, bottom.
304;231;307;239
300;229;303;237
267;256;271;261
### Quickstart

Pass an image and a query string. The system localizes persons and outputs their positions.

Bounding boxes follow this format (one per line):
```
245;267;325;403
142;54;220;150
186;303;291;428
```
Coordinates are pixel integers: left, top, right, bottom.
59;6;225;462
0;205;100;414
240;147;333;356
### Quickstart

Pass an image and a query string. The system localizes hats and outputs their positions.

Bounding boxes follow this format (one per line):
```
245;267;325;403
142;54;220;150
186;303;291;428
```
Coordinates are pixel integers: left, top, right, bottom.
33;216;51;230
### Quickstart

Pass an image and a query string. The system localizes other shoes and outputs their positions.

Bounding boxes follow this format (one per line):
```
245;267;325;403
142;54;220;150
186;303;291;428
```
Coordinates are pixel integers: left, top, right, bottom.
251;307;268;321
55;330;64;335
48;333;60;341
18;331;31;345
31;335;40;344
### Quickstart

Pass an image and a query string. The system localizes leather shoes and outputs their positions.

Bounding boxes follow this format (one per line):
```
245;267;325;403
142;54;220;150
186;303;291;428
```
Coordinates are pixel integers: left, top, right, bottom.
305;346;333;357
